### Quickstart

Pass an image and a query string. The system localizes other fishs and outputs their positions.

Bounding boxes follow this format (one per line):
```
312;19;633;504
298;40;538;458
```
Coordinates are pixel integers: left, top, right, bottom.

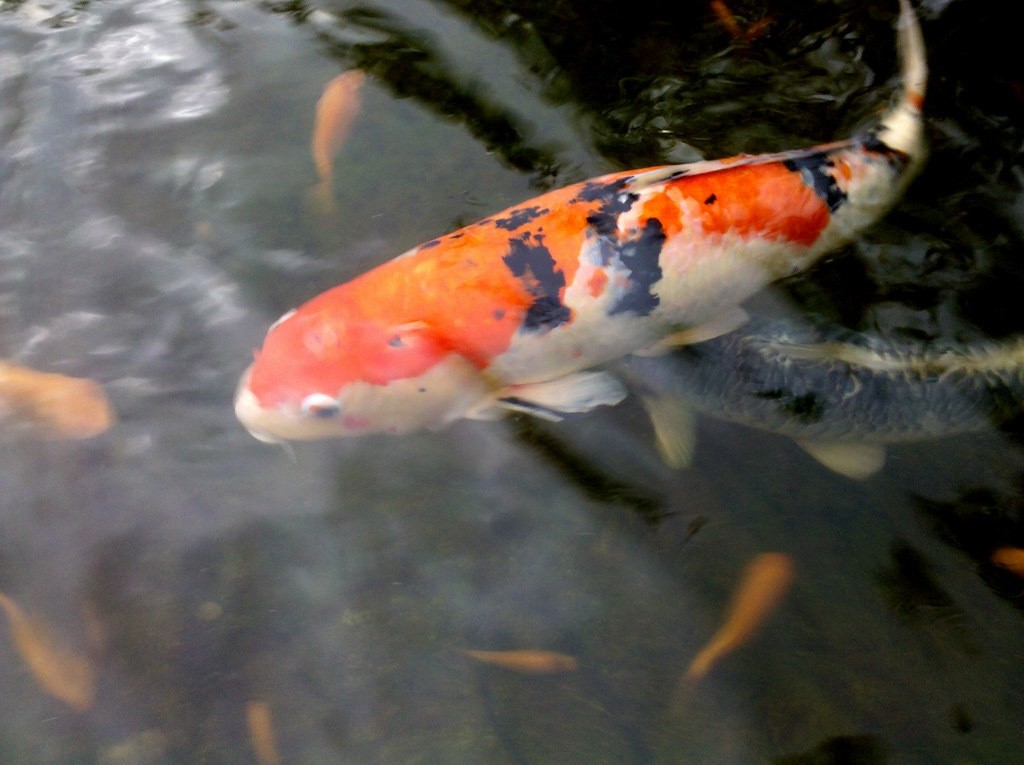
995;548;1022;575
0;593;276;765
234;0;1024;478
465;647;582;678
684;551;792;676
0;362;117;446
